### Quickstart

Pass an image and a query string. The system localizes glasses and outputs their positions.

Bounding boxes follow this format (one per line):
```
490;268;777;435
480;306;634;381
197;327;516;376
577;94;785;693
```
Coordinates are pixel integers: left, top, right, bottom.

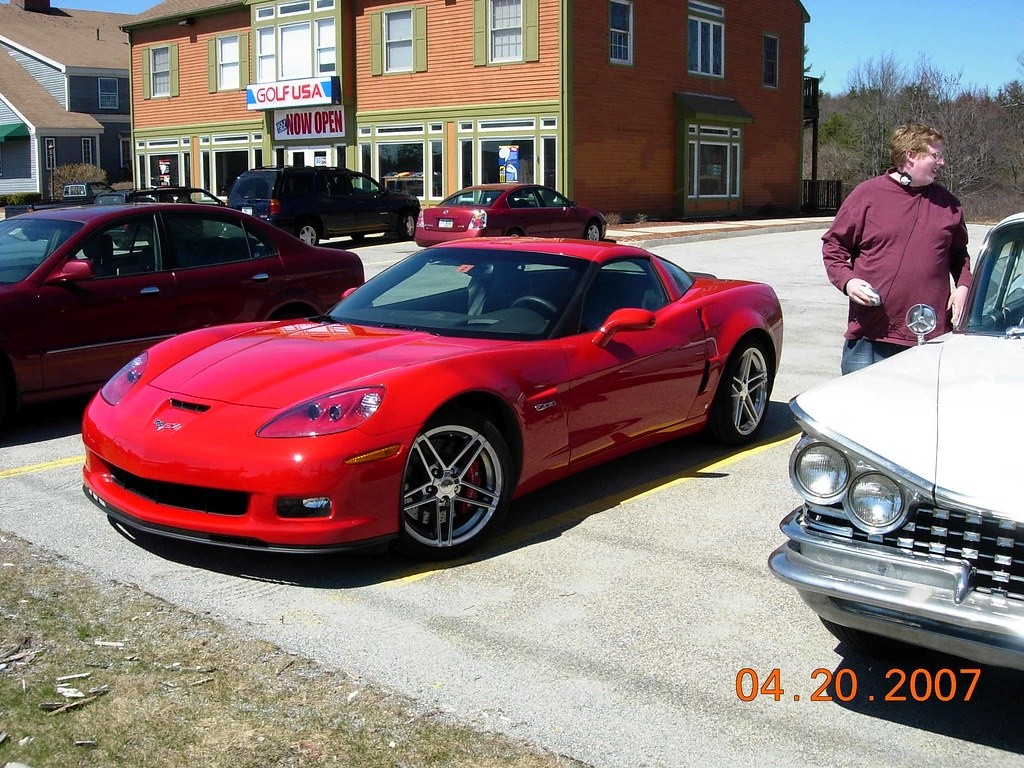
912;149;945;161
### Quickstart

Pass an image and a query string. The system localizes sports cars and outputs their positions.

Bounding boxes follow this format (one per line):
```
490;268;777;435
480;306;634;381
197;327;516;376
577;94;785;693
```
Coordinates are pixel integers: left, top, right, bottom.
81;236;784;559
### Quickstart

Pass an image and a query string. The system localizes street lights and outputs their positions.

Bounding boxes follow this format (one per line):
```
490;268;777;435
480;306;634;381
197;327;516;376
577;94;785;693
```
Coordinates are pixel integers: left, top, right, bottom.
49;142;54;202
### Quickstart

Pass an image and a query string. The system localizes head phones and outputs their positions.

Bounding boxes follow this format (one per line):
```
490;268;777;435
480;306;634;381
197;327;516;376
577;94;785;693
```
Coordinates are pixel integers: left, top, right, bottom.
896;170;912;186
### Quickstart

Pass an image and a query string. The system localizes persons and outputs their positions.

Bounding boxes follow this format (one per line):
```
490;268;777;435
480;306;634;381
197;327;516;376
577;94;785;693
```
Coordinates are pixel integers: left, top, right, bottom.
822;120;972;377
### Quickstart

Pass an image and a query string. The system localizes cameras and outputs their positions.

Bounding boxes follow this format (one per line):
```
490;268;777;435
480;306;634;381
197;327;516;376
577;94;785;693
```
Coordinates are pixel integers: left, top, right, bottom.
861;285;881;306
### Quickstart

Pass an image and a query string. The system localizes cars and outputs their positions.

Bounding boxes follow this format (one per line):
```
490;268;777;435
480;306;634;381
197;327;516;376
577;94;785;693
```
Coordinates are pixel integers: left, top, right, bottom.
415;183;607;247
0;203;366;422
768;211;1024;672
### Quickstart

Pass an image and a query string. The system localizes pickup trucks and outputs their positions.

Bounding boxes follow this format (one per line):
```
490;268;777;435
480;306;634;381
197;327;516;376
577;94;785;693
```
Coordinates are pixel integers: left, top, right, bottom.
5;182;116;218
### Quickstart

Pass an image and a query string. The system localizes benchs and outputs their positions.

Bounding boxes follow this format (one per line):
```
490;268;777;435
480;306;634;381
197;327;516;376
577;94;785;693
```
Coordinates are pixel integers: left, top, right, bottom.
171;237;261;269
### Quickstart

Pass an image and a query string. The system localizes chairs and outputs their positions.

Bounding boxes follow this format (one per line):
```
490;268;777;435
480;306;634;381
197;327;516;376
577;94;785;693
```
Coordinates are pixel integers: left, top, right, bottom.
161;194;174;203
468;264;538;316
553;265;607;334
176;193;192;203
82;233;117;277
507;192;516;208
141;230;175;271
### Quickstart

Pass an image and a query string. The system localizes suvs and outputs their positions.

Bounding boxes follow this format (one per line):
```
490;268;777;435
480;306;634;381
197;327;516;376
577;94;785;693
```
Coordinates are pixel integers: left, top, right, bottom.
95;185;227;248
227;165;421;247
